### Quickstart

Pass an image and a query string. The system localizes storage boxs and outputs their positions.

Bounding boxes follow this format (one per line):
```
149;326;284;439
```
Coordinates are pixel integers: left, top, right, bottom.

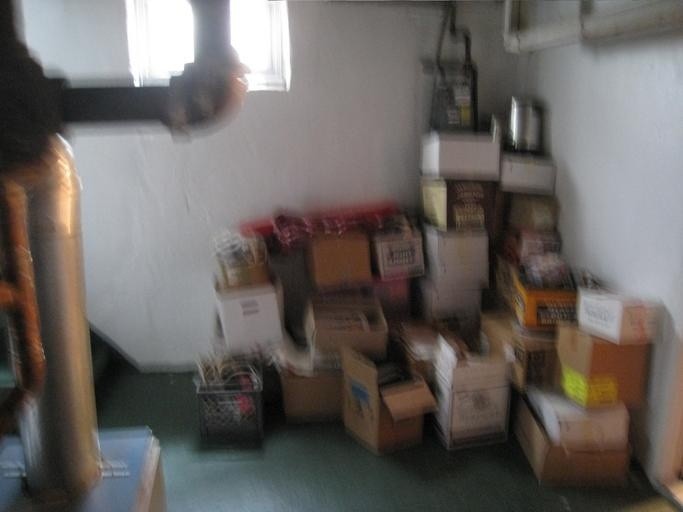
399;287;662;490
194;199;425;454
417;130;567;287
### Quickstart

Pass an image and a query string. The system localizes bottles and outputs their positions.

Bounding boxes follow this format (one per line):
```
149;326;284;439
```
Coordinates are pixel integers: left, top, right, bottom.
509;97;541;153
